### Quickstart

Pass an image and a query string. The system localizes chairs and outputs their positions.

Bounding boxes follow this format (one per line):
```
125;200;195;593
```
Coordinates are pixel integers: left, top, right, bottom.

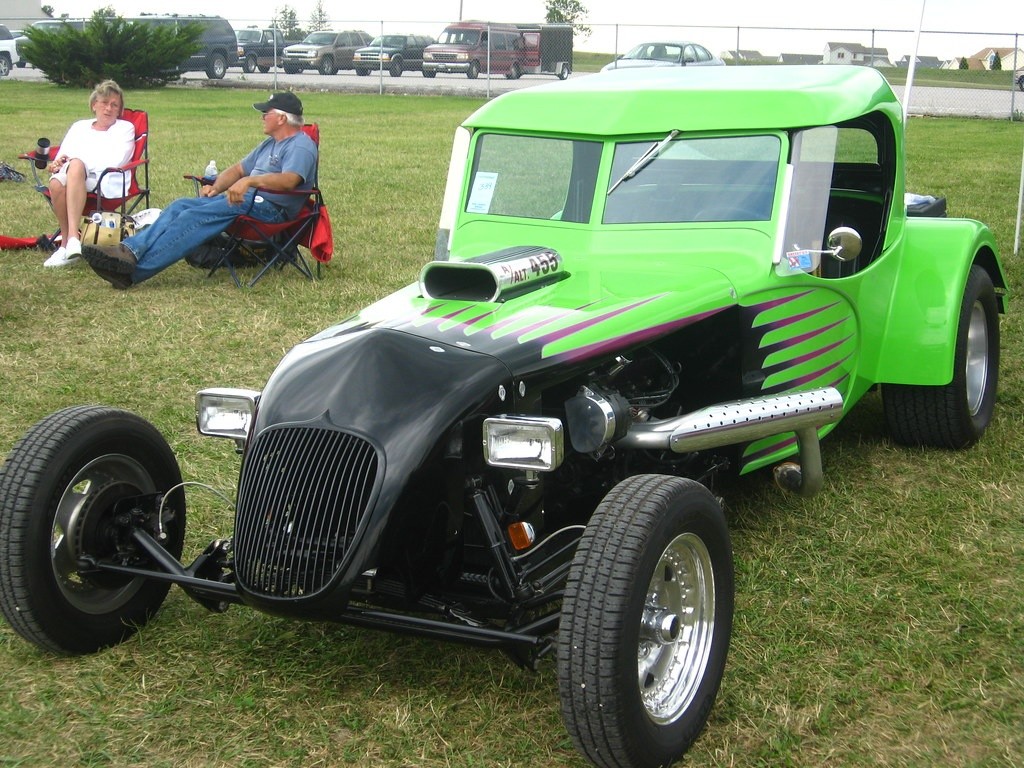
19;106;149;251
183;124;321;289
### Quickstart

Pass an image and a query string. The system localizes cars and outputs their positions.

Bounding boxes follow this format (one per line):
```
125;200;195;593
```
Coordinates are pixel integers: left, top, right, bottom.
599;40;728;71
0;14;240;77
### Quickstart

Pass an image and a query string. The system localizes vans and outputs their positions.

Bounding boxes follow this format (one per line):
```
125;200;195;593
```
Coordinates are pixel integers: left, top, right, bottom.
513;23;574;80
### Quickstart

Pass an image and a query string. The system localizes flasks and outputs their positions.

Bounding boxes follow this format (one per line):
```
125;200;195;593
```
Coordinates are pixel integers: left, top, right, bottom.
35;138;50;169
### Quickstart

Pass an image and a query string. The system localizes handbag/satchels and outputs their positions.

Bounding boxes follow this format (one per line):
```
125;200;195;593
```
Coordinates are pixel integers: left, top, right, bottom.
79;210;136;246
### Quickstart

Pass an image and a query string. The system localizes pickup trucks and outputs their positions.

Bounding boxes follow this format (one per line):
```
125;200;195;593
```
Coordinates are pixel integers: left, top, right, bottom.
232;27;304;74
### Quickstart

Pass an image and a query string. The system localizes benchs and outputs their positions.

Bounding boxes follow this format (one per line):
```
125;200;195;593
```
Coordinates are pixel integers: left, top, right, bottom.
637;185;887;278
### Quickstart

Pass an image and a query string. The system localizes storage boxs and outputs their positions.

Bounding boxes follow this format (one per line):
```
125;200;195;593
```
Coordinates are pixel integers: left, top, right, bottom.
222;232;277;265
907;197;948;218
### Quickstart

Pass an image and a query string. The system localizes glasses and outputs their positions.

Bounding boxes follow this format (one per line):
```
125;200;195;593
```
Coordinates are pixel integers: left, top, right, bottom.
261;110;275;118
95;99;121;111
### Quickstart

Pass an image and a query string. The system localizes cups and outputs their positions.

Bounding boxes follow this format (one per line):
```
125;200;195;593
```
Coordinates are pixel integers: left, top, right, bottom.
92;212;102;223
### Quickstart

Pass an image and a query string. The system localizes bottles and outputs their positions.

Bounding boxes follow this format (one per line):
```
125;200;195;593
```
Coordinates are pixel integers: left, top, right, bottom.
205;161;218;180
105;213;116;229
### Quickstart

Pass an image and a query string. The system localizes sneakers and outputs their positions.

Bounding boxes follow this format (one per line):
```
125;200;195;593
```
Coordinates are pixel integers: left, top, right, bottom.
64;236;82;260
81;246;136;275
90;264;133;290
44;245;78;267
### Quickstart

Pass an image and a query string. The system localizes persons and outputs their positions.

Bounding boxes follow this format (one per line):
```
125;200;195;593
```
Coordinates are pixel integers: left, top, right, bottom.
80;90;318;291
44;79;135;267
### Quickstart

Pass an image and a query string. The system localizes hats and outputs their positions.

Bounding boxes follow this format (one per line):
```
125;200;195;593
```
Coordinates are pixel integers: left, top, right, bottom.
253;91;304;115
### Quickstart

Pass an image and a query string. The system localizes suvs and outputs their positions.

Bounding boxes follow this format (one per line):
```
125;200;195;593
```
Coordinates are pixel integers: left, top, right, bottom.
353;33;438;78
0;63;1011;768
281;28;376;75
421;19;528;82
